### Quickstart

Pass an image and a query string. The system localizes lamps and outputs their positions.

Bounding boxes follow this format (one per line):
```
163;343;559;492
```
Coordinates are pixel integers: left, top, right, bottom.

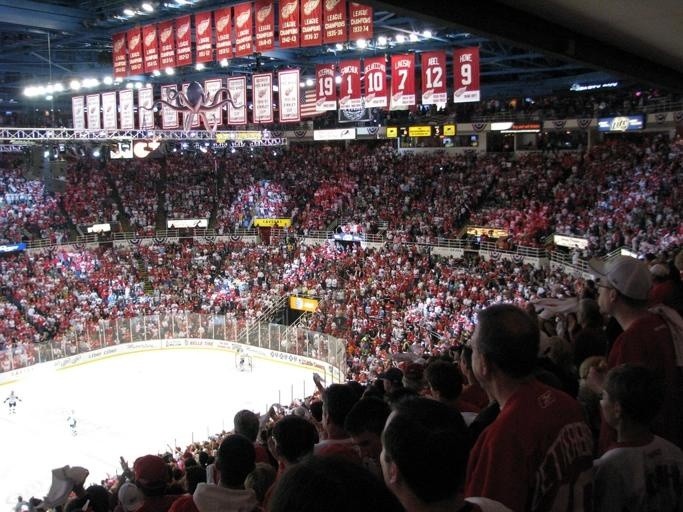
141;0;159;12
124;6;137;16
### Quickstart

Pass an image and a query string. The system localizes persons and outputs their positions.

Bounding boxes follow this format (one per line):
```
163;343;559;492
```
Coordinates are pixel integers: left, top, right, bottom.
1;86;682;512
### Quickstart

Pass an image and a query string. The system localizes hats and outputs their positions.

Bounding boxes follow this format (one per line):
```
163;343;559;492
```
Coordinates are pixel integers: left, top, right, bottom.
375;363;424;380
132;453;169;480
587;250;657;302
117;481;147;512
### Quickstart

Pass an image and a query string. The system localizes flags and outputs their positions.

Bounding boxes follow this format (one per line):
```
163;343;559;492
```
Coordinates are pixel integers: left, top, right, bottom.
204;79;222;125
250;72;274;125
158;19;175;69
453;47;480;104
277;0;299;49
348;2;373;39
214;6;233;61
362;56;388;108
277;67;302;124
225;75;246;124
338;58;361;111
419;50;447;111
255;0;273;51
315;63;338;111
111;32;128;77
175;15;192;67
298;0;323;47
142;24;159;72
160;83;180;129
182;82;201;128
387;53;416;111
323;0;347;44
127;28;144;76
194;11;213;63
233;1;254;57
